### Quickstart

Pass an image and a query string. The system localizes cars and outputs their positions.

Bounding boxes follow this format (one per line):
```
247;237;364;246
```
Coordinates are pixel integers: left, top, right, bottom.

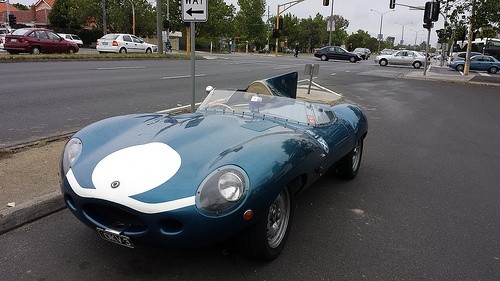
353;47;371;60
449;55;500;75
314;46;362;63
57;72;368;264
453;52;482;61
258;49;270;54
96;34;157;55
374;50;431;70
0;28;16;38
3;28;79;54
379;48;449;62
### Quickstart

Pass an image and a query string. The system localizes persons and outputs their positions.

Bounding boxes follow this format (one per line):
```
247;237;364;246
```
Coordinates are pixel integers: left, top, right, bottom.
294;42;299;58
347;44;352;52
165;39;172;53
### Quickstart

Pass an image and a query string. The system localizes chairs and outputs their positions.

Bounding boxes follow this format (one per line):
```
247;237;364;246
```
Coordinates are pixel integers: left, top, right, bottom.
247;81;273;96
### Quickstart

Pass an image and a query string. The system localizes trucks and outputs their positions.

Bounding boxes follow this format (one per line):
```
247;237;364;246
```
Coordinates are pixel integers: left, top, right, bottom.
54;33;83;47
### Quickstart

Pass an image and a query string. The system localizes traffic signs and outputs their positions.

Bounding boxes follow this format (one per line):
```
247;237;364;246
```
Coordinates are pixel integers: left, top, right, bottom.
182;0;208;22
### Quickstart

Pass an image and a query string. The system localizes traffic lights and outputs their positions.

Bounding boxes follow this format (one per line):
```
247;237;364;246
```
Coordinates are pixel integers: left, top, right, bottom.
9;14;16;26
273;29;280;38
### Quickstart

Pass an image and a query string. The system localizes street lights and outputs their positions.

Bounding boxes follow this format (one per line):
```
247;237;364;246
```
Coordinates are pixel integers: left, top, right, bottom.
394;21;415;51
370;9;395;54
410;28;423;49
